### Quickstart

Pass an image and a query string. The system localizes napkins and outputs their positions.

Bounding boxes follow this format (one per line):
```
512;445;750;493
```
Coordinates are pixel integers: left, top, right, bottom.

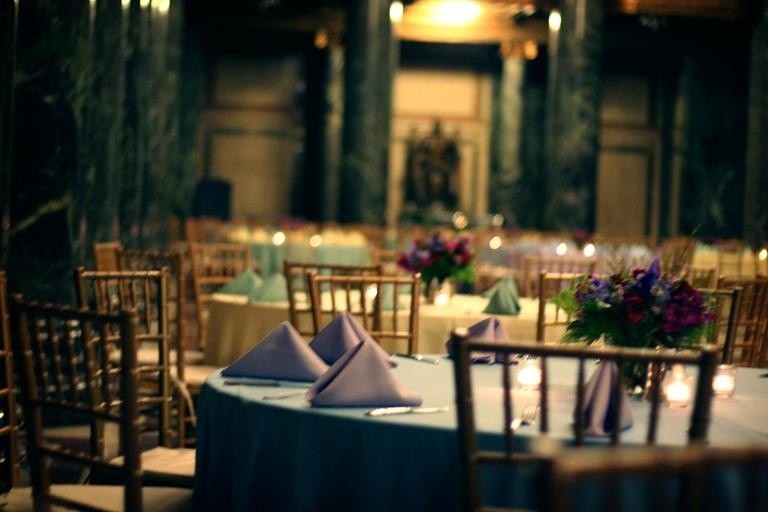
306;336;426;410
583;359;635;437
220;322;330;383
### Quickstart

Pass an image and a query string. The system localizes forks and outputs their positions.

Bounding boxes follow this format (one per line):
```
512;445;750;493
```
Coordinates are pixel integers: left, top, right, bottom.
509;404;538;432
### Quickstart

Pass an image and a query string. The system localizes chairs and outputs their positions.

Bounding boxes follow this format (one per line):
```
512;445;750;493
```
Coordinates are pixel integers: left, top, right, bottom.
1;270;121;510
74;265;173;448
0;215;768;366
448;327;719;510
6;293;195;509
114;248;220;448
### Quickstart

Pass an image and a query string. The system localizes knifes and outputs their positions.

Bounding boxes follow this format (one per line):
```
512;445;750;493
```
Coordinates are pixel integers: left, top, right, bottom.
365;405;445;418
